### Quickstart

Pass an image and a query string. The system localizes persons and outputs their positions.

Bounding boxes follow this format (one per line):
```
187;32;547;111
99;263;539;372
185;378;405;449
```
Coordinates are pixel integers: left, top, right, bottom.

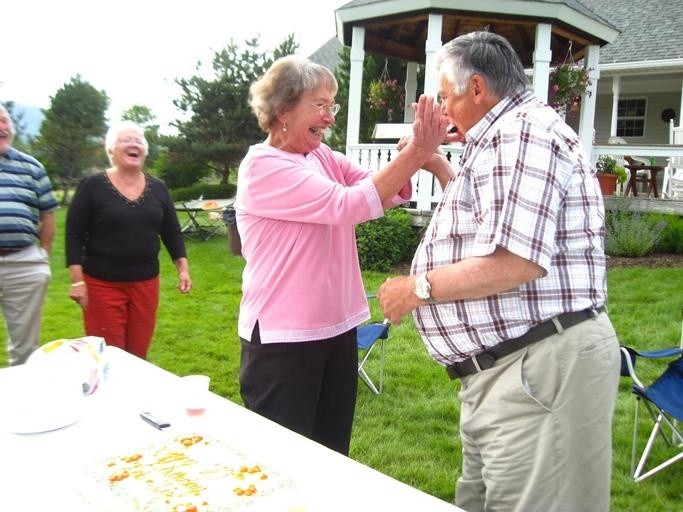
232;54;449;457
1;104;61;367
375;31;622;511
64;120;192;360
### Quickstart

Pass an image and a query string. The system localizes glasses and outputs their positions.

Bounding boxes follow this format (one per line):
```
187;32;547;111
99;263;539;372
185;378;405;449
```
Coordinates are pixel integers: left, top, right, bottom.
310;103;341;117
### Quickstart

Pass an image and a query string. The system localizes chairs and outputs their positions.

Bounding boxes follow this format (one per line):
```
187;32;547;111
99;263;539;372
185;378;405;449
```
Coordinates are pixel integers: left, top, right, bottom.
357;293;390;394
620;346;682;485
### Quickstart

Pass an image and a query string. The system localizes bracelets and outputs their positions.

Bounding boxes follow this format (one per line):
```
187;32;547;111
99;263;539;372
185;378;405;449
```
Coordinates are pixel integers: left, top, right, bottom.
71;281;85;287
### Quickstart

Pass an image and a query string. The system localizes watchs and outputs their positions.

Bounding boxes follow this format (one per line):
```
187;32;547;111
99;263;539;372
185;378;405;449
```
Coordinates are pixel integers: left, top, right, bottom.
413;271;438;305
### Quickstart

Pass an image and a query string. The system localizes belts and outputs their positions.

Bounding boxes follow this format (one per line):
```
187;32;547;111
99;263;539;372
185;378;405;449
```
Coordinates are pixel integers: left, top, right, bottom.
445;305;609;379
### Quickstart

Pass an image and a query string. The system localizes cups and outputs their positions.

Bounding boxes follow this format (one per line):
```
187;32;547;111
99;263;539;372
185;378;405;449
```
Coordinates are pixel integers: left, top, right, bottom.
178;373;212;418
649;156;656;166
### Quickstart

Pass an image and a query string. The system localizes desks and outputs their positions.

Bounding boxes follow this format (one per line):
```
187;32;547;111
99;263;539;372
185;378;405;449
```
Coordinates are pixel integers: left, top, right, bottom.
624;164;664;198
173;195;235;243
1;344;464;512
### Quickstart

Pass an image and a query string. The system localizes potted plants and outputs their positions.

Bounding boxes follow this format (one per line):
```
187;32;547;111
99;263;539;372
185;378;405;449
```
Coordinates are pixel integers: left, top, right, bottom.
548;39;594;116
367;58;405;113
595;155;626;196
